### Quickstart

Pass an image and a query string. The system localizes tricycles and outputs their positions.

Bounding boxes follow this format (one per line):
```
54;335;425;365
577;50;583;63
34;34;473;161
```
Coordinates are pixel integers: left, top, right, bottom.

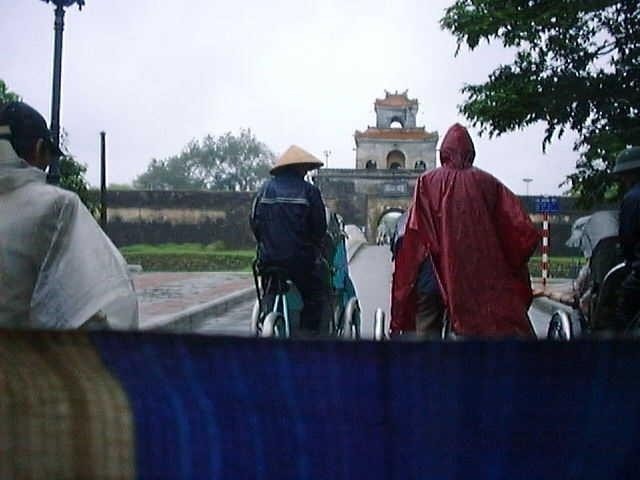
541;210;633;340
249;211;361;341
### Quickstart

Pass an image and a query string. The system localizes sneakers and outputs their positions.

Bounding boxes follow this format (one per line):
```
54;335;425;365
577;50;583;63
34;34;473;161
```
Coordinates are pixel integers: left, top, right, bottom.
299;328;321;336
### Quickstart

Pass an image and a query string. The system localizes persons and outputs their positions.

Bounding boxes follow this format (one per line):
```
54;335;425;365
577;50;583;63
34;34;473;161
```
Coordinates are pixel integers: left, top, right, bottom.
607;142;640;338
388;122;539;341
375;223;387;247
247;143;330;338
0;98;140;331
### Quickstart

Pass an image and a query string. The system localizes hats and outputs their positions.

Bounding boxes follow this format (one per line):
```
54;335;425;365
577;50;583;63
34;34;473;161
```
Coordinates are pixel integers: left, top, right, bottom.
270;145;323;175
613;146;640;173
0;101;67;159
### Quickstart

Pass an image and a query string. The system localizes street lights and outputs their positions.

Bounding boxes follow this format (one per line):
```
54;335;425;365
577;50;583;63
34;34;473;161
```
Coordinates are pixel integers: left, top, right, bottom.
39;0;88;187
520;178;534;196
